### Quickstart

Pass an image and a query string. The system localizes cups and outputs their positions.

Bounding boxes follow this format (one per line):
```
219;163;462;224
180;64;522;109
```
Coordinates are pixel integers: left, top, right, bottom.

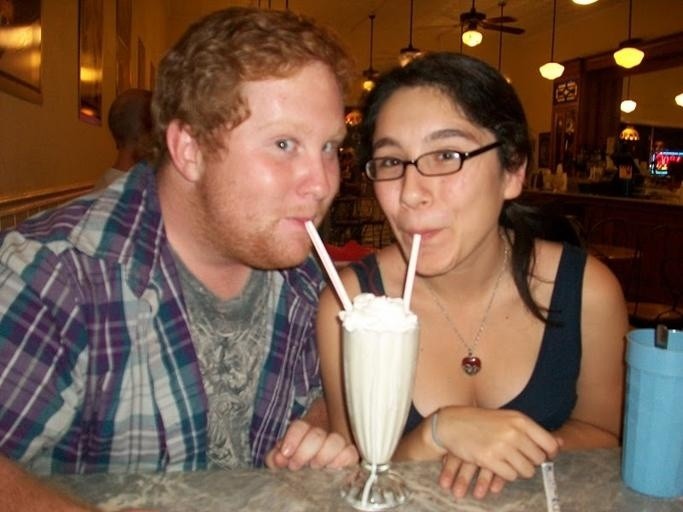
621;328;683;499
339;298;419;512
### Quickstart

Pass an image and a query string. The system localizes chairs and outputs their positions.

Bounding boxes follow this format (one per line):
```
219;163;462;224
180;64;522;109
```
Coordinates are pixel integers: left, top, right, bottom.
581;216;683;329
330;195;385;250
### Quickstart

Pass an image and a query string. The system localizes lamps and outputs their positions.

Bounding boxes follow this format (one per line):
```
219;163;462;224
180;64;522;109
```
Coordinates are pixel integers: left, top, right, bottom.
620;73;641;142
360;0;646;93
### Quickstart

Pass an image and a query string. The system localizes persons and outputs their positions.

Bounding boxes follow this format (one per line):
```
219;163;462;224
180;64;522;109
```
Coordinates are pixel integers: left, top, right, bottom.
0;6;362;512
99;88;156;192
314;50;633;502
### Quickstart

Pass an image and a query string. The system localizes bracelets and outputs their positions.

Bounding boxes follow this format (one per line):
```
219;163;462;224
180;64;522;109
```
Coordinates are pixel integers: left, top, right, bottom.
431;405;446;452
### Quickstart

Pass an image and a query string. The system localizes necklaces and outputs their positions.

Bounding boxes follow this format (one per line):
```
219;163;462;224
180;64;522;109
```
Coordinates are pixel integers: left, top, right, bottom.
420;230;509;376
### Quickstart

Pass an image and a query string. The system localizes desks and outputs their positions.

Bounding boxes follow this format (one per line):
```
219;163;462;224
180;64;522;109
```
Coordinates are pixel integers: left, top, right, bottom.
37;445;682;511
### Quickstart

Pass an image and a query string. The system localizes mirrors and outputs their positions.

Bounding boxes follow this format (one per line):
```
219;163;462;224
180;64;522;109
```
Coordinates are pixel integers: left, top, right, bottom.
593;53;683;181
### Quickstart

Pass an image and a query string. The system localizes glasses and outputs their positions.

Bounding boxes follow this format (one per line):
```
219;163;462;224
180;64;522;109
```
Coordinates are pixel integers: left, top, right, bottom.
363;140;502;182
